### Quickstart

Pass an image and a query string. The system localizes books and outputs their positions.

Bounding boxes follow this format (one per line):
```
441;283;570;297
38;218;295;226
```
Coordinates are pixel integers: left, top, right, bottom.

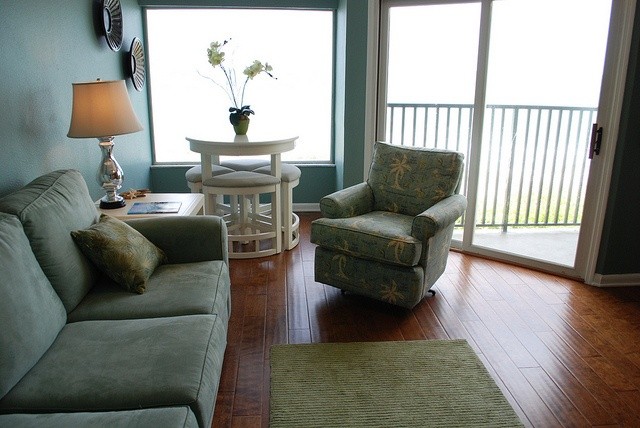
128;202;182;214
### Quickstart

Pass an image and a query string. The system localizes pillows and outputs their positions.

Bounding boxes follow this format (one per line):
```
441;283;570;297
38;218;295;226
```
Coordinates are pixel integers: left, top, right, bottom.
67;210;169;295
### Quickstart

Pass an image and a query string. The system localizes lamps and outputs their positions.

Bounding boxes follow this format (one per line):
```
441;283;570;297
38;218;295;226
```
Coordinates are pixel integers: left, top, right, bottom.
67;76;144;211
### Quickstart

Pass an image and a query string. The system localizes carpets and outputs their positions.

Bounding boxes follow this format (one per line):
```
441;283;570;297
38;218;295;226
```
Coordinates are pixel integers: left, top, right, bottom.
268;337;527;428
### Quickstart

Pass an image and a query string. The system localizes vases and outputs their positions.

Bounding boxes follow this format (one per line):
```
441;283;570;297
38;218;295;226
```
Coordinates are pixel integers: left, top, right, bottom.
231;119;251;135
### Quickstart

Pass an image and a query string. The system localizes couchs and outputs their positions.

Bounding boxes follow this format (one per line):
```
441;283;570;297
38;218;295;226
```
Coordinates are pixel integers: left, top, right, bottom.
0;168;235;428
309;138;469;312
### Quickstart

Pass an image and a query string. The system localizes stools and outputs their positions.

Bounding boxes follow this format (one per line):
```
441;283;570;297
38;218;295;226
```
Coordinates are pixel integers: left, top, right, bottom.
217;156;275;243
252;162;302;253
184;160;239;261
201;169;283;260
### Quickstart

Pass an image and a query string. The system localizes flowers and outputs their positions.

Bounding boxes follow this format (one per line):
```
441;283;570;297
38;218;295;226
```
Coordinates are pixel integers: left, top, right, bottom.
206;35;278;126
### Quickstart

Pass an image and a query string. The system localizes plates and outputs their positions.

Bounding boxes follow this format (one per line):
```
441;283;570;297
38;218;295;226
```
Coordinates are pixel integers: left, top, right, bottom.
128;37;144;92
98;0;123;51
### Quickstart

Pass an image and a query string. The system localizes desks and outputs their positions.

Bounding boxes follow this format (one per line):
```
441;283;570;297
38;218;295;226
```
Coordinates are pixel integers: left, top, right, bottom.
183;130;300;260
90;191;207;221
